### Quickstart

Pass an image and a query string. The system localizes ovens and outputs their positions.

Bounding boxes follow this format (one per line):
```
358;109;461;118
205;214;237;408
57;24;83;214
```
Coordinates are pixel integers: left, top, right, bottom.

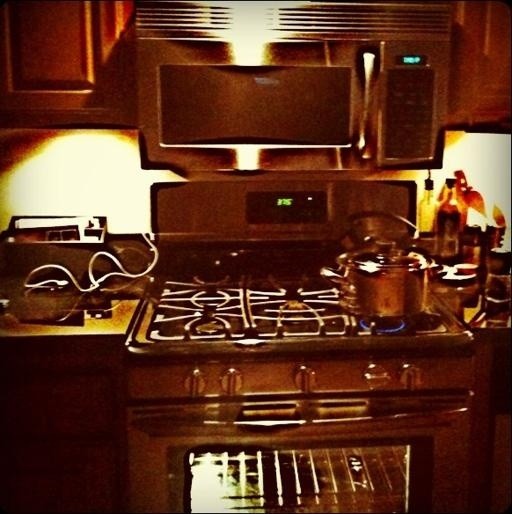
125;347;494;512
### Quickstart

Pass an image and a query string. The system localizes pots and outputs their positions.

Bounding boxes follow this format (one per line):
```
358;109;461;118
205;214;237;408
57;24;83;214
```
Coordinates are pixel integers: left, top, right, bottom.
320;241;442;322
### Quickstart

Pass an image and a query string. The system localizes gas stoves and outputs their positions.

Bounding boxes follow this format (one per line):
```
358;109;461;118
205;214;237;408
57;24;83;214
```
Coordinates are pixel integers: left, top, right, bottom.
124;264;478;344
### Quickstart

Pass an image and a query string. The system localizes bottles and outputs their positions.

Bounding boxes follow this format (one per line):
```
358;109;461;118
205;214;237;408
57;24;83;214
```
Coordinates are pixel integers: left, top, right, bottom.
420;179;511;322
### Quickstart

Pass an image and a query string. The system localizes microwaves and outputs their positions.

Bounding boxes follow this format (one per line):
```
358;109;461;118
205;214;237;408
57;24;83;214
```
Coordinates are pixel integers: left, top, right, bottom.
136;33;453;171
